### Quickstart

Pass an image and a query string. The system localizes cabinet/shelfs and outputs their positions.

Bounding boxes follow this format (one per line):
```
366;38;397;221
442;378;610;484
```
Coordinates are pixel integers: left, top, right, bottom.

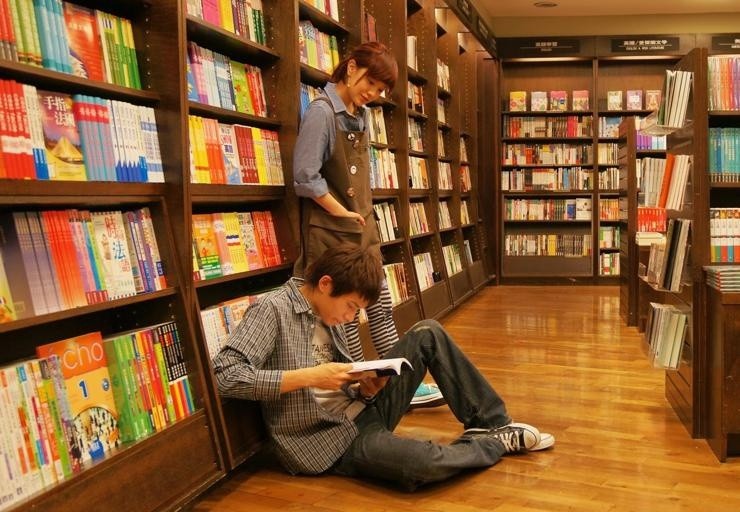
182;1;301;494
0;1;228;512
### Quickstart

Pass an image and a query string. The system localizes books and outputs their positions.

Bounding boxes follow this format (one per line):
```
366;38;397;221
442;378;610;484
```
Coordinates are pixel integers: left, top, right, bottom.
193;211;281;281
200;287;271;363
2;76;166;183
1;320;196;509
634;53;740;372
351;358;415;376
2;0;142;91
300;0;378;125
0;205;169;325
185;0;287;186
499;89;633;276
367;36;475;308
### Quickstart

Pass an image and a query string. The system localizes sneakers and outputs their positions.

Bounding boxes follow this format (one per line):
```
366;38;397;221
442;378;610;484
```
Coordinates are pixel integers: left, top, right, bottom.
408;382;448;408
528;433;555;451
463;423;540;455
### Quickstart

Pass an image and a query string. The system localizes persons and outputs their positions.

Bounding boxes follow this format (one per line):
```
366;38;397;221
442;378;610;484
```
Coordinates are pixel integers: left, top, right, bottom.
210;240;557;496
292;42;444;405
